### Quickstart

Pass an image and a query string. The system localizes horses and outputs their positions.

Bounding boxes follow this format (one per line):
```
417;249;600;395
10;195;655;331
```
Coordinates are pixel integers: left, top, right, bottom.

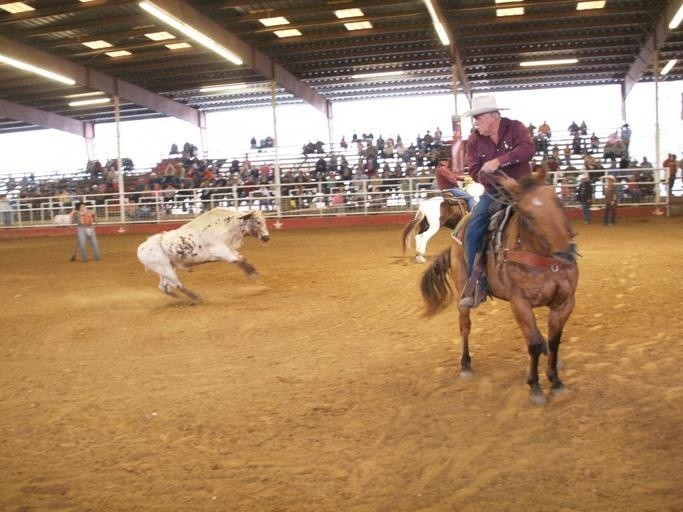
419;158;579;406
400;181;485;265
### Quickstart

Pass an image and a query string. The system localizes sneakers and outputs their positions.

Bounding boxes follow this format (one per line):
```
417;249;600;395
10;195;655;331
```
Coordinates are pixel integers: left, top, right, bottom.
459;286;488;308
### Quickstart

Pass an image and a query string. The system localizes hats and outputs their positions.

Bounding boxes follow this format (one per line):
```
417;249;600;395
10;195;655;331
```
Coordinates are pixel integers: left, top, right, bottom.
580;175;589;180
436;153;453;161
462;96;511;118
607;175;615;183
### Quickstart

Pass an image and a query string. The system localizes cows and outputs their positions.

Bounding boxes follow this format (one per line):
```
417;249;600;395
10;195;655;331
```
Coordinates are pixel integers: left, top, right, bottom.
136;207;271;302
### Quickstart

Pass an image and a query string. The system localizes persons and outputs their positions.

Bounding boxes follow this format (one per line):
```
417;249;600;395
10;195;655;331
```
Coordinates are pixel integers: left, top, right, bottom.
279;127;469;218
121;136;276;224
458;95;578;310
6;159;121;224
436;152;476;212
527;120;682;224
69;202;102;262
